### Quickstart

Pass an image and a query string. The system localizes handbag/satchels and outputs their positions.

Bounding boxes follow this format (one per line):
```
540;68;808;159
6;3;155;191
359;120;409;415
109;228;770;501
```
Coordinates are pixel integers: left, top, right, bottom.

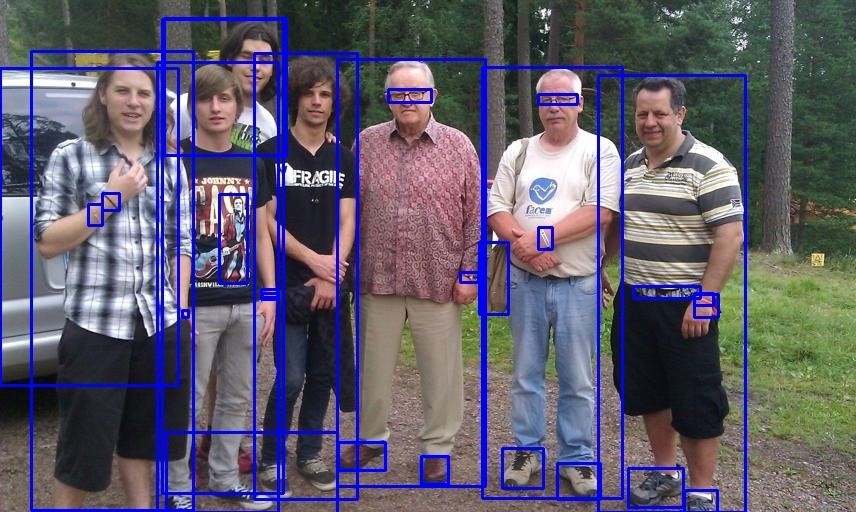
479;243;509;314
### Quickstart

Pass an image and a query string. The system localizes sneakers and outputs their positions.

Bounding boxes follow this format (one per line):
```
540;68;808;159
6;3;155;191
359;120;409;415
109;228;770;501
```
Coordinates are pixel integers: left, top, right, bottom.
685;491;717;512
557;464;601;500
502;448;544;489
166;432;338;512
629;468;683;507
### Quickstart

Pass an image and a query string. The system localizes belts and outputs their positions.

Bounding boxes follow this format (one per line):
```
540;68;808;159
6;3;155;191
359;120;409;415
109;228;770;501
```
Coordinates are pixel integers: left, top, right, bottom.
634;286;700;298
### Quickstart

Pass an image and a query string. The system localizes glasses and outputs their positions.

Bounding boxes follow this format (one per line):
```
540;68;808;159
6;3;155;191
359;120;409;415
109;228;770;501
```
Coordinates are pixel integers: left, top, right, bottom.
388;89;432;103
539;95;578;104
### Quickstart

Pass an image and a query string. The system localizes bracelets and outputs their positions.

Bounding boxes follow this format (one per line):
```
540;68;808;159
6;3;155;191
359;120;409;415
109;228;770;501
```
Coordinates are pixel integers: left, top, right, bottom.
539;228;552;249
694;294;719;317
183;310;189;317
460;272;479;282
88;204;102;225
262;293;278;297
102;193;119;210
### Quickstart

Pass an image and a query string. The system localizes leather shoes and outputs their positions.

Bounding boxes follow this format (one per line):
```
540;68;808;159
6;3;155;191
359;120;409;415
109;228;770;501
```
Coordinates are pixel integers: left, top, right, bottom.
422;457;449;485
339;442;386;470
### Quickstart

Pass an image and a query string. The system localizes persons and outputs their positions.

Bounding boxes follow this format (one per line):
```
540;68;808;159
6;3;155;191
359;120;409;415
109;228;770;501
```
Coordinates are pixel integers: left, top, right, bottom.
219;195;248;283
254;54;358;500
599;76;745;512
338;59;486;487
32;51;194;510
162;20;283;493
483;67;623;499
158;62;278;512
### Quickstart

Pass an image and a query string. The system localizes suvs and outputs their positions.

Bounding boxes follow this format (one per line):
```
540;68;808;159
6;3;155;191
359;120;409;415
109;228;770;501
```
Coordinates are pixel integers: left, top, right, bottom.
0;68;179;385
